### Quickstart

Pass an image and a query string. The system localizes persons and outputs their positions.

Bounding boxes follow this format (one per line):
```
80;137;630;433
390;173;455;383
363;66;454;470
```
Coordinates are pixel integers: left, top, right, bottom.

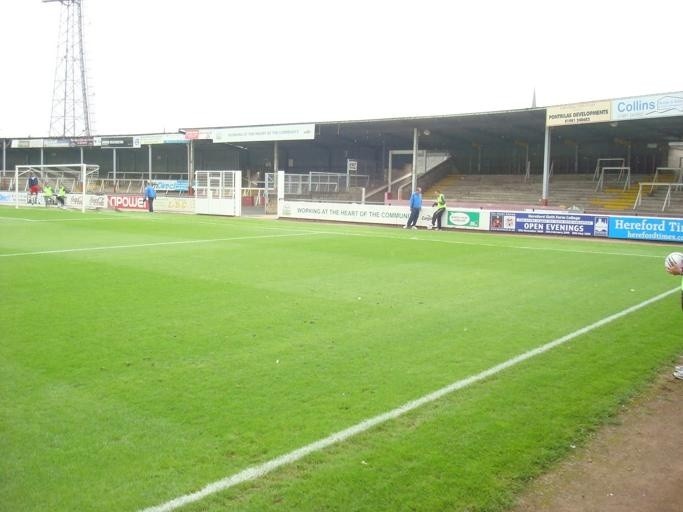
56;181;65;209
432;190;447;232
666;254;683;379
144;181;157;213
42;182;53;208
28;171;39;205
403;187;423;231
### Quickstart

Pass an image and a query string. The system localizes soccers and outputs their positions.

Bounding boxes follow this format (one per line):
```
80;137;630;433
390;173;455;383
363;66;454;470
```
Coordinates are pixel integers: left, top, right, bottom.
665;251;683;271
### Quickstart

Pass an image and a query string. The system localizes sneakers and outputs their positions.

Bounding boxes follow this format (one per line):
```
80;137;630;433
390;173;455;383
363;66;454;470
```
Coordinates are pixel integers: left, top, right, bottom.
403;225;417;230
672;365;683;379
435;228;442;231
